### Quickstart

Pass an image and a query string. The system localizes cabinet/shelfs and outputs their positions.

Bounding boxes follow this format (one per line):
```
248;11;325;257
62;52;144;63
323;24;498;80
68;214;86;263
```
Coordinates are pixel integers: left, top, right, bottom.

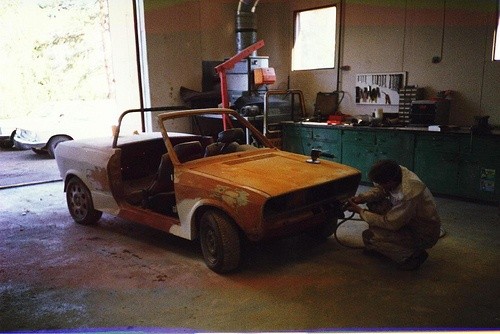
279;117;500;202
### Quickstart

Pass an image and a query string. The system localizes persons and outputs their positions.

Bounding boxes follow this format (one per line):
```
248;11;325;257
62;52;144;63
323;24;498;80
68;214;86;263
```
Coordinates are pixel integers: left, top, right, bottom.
344;159;443;272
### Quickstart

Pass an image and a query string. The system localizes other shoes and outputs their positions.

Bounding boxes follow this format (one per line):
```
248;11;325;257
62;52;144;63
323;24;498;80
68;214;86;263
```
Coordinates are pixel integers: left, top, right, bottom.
407;251;430;273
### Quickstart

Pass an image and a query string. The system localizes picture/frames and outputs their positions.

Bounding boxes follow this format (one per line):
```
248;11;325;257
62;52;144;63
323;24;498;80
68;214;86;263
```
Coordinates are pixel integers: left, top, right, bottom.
355;70;408;105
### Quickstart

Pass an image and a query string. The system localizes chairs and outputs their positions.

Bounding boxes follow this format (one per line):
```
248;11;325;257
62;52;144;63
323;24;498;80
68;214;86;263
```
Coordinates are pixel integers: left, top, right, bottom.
204;128;245;158
143;141;204;208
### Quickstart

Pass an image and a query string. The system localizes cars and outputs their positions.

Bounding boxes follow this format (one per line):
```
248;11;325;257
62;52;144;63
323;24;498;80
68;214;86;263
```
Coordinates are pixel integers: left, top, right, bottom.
55;104;365;275
0;96;144;159
